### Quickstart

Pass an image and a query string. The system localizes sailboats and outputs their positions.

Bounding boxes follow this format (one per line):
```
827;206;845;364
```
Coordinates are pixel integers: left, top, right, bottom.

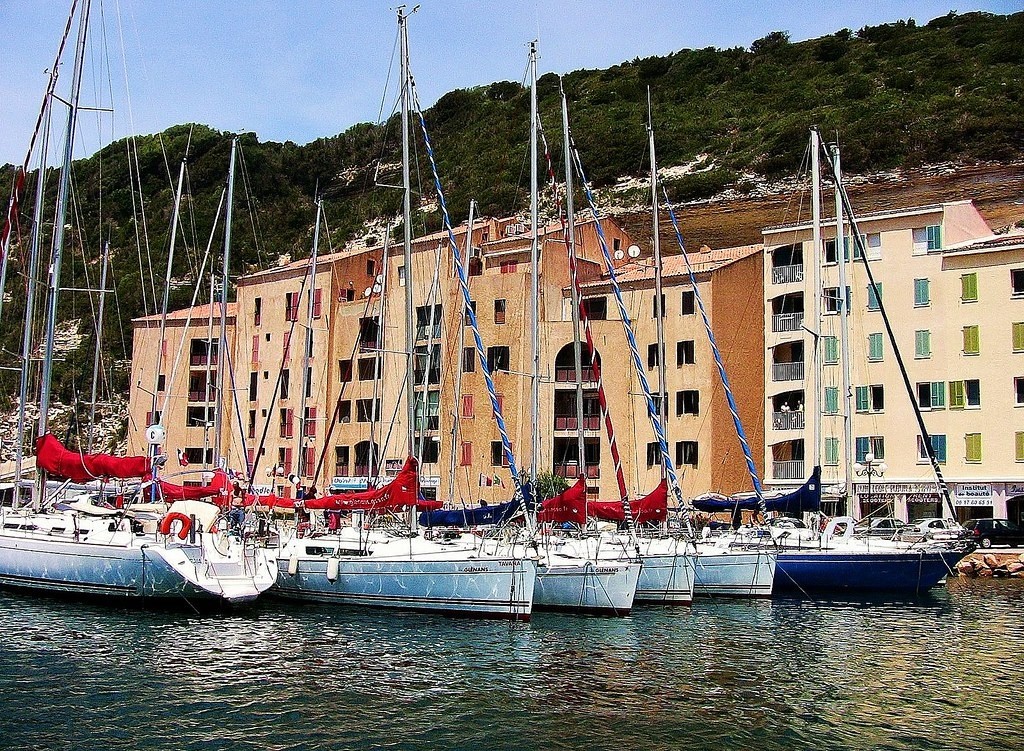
0;1;978;620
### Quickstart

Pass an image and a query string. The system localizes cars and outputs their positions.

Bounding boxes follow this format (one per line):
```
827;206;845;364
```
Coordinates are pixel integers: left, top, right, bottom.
896;518;965;539
853;516;906;536
961;518;1024;549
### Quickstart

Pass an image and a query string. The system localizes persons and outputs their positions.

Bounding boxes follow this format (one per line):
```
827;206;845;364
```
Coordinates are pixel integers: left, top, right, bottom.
781;402;791;412
798;401;803;411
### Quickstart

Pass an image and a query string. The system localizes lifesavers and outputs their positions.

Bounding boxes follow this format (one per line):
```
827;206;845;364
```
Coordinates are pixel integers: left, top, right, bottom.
161;511;193;540
298;522;312;538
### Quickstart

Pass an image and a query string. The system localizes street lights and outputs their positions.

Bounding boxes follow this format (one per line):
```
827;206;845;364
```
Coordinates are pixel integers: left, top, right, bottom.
853;453;887;551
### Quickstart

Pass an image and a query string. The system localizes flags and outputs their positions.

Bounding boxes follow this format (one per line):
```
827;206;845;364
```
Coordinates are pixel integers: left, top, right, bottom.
178;448;189;467
